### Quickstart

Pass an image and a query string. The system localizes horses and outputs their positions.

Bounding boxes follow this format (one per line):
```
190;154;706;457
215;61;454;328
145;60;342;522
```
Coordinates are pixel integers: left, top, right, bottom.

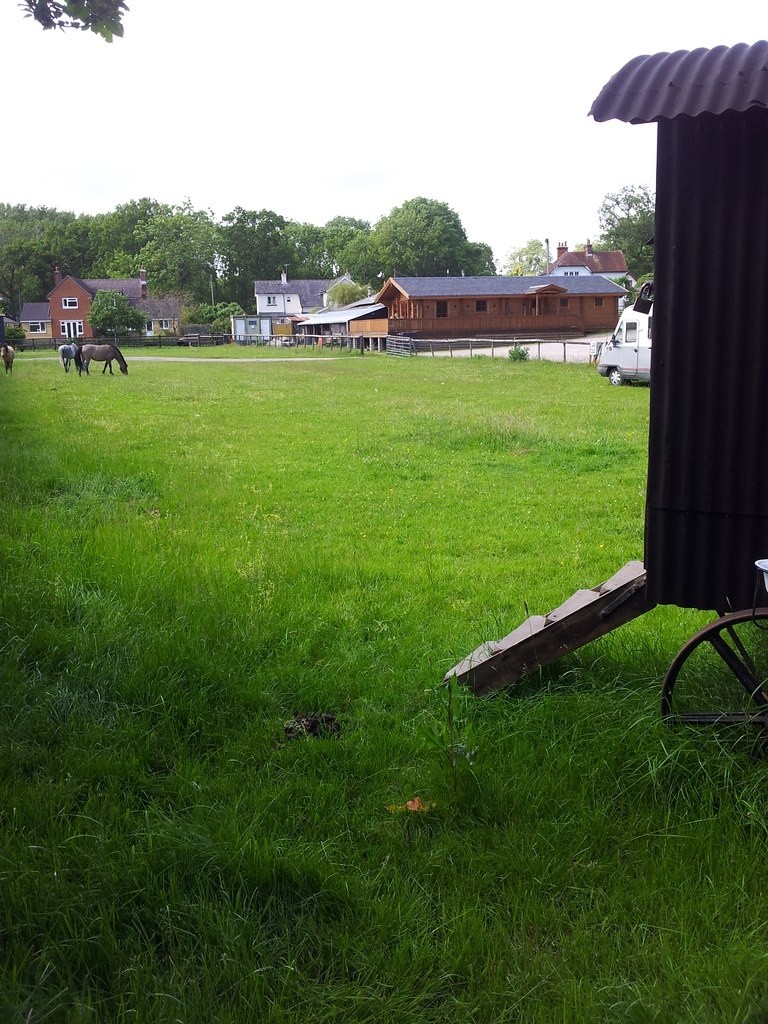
59;342;78;373
73;344;128;375
0;343;16;374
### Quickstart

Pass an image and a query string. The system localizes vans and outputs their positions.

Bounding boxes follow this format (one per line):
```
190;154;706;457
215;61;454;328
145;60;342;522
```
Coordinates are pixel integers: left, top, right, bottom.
596;304;653;386
